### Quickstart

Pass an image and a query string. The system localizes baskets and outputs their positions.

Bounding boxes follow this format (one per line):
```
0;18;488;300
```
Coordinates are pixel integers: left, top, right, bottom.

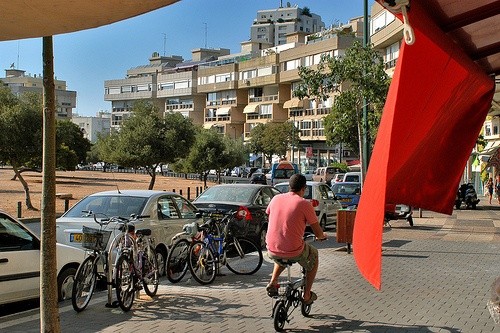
230;216;249;238
81;225;112;252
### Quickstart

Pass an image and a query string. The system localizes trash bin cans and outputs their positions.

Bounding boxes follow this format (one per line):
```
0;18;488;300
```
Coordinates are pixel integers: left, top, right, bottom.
336;210;356;253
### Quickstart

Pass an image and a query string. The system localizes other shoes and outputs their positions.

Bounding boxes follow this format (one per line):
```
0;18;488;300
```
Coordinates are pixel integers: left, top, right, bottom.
266;282;279;299
303;291;317;305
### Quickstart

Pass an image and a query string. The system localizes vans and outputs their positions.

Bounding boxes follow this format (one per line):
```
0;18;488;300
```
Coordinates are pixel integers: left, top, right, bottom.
311;167;341;184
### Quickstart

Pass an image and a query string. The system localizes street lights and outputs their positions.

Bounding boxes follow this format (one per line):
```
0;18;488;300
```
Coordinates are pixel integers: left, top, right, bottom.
289;114;295;163
230;127;236;141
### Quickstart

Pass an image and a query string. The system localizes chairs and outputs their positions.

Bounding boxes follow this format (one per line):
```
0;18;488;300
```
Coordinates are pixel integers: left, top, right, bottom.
353;189;360;194
340;189;346;194
88;202;171;218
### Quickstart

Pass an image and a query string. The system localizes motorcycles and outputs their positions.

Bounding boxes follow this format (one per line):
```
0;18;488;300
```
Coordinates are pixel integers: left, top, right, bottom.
458;182;480;210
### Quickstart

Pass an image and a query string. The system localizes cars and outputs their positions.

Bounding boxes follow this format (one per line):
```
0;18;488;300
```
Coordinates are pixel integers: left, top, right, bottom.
209;167;272;185
328;182;361;209
302;171;314;181
331;173;345;187
341;172;361;193
155;164;168;173
183;183;283;251
272;181;340;231
55;190;203;280
0;210;91;307
93;162;105;169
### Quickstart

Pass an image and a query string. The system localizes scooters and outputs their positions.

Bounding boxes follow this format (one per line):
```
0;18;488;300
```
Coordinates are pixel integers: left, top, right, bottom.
385;205;414;228
268;234;328;331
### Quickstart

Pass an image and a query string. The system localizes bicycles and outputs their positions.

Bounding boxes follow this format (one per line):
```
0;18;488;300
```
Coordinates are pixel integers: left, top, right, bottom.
165;209;263;285
101;213;159;312
72;210;135;313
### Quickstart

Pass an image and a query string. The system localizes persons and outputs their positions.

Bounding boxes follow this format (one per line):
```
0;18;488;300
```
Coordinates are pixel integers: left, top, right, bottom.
486;175;500;205
265;175;326;305
